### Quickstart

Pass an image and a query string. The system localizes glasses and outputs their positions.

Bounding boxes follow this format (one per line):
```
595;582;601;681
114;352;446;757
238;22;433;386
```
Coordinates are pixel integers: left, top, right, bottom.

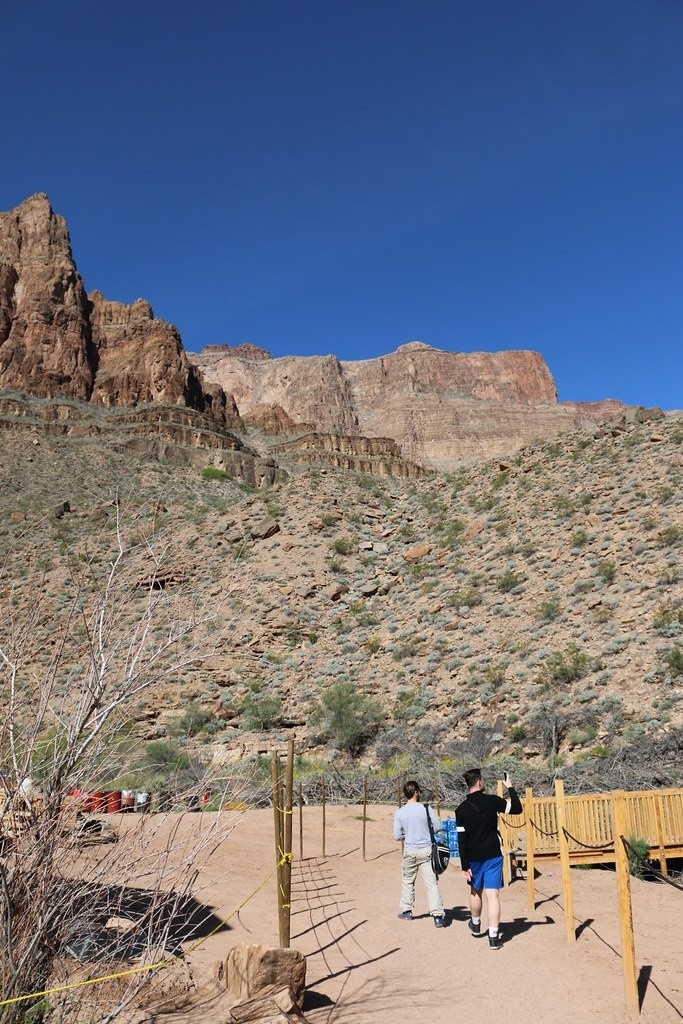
419;789;422;793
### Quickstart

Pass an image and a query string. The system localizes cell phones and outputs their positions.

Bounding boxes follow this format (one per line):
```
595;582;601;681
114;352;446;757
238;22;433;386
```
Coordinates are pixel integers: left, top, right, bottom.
503;771;507;781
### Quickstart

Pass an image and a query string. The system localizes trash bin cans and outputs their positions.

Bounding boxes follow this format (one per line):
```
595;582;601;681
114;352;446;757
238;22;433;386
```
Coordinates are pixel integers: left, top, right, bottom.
68;791;168;812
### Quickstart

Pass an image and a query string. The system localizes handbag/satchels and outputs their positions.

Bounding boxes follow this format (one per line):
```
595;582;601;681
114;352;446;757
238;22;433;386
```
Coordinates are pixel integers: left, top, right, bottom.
431;842;450;874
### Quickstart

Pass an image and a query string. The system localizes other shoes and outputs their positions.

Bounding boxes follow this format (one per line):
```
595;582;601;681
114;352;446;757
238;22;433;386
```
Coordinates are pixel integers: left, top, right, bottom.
434;916;446;927
487;931;500;949
398;911;412;920
467;919;481;935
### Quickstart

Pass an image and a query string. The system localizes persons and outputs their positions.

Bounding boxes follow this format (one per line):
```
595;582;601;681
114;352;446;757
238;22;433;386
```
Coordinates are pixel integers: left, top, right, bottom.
394;781;446;927
454;768;523;950
